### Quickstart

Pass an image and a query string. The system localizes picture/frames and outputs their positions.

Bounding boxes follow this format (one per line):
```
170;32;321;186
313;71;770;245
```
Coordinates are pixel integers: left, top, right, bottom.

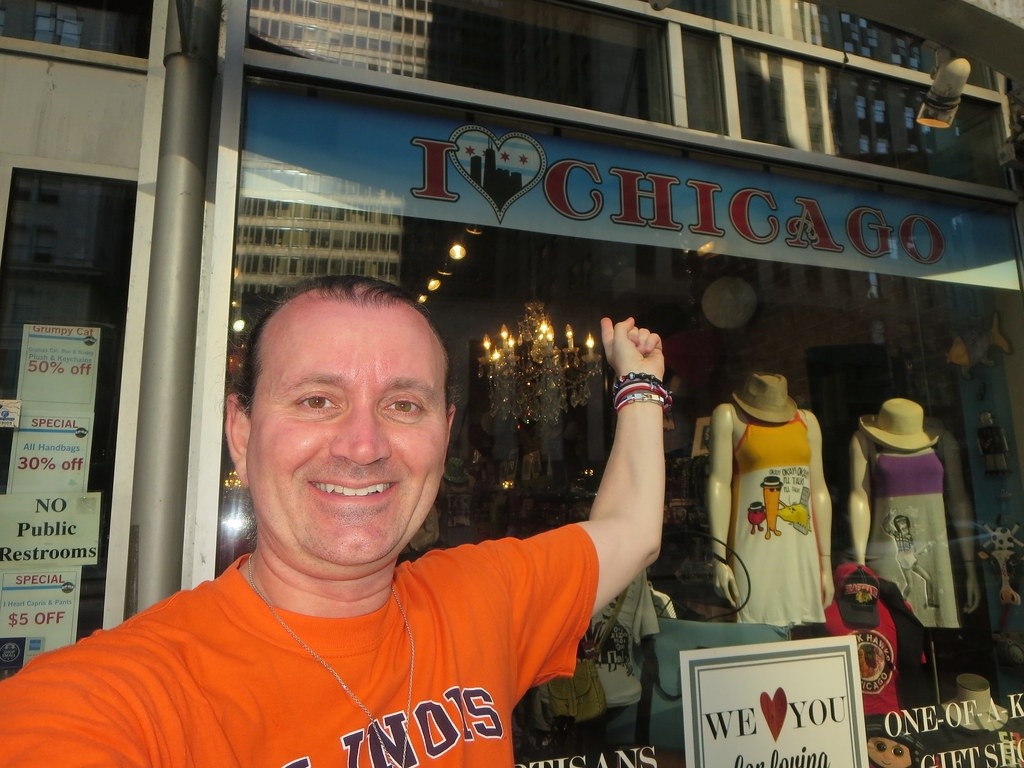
506;448;519;482
498;459;508;483
690;415;711;457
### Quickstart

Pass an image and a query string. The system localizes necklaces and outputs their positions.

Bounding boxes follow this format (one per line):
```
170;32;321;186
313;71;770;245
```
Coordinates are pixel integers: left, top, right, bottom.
248;553;414;768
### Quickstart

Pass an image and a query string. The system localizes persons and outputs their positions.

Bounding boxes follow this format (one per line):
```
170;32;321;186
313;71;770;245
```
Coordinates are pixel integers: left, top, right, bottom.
707;372;836;625
0;275;665;768
847;398;980;704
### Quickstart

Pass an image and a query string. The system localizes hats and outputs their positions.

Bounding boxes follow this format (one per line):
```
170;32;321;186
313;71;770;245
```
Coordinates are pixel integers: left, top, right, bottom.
443;456;468;483
733;370;797;424
835;562;880;626
521;497;545;519
859;398;945;452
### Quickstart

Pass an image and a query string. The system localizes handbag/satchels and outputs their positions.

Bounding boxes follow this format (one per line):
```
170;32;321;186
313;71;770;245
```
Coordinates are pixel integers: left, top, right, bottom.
546;659;608;725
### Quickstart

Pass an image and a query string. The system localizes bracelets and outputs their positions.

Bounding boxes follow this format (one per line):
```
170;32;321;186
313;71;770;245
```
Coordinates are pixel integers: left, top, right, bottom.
612;371;673;415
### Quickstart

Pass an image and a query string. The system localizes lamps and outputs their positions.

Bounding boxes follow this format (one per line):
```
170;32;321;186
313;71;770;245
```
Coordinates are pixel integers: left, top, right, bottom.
917;49;972;130
479;236;604;427
415;224;484;304
